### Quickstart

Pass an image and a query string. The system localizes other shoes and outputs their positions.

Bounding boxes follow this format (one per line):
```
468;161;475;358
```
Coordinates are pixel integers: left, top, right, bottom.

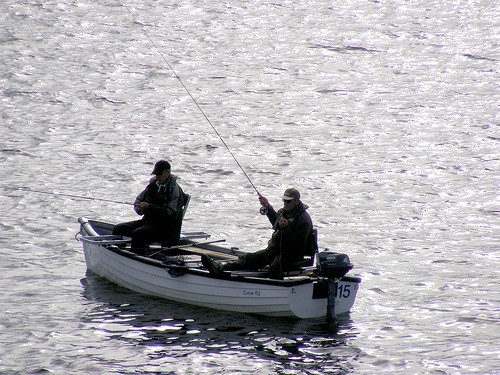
201;254;223;277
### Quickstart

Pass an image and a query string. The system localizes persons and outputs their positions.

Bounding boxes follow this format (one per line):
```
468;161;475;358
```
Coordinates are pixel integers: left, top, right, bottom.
201;188;314;280
112;161;184;256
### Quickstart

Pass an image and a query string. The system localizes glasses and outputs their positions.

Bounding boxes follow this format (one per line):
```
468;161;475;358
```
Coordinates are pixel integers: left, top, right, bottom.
284;199;292;203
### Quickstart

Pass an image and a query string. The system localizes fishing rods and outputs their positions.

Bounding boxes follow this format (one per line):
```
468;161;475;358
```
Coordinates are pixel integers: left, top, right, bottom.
6;188;143;210
125;6;277;215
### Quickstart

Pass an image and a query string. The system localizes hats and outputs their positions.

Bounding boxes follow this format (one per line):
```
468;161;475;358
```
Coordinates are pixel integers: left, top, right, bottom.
151;160;170;175
282;188;300;200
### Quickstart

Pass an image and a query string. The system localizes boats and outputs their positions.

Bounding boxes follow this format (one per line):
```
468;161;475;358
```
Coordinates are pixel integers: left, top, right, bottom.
75;216;361;319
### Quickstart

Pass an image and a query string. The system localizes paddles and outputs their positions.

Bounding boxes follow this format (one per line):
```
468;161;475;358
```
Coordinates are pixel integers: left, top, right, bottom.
149;239;225;258
